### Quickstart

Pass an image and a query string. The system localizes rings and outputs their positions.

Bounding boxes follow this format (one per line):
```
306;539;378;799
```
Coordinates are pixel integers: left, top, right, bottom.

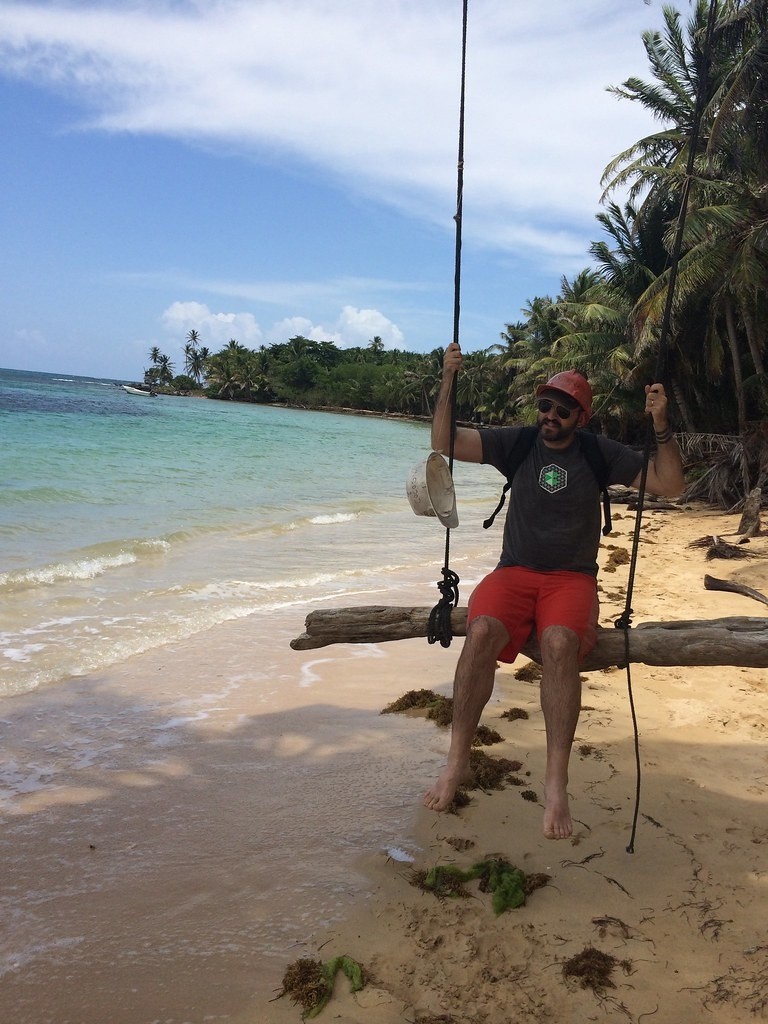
651;401;654;405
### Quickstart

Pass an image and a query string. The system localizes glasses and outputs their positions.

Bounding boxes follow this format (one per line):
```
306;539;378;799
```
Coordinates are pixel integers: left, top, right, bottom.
539;401;570;419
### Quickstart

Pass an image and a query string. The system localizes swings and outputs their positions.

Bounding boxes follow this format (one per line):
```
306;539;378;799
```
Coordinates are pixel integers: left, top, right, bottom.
288;0;768;665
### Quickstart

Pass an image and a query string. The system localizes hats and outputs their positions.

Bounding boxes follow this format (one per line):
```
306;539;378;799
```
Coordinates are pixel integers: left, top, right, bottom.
406;451;460;529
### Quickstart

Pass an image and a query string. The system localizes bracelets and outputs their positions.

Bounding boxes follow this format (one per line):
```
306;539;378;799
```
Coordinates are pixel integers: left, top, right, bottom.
653;426;673;444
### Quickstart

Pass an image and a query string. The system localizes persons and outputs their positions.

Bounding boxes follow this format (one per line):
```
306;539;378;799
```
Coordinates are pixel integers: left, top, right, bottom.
421;342;690;838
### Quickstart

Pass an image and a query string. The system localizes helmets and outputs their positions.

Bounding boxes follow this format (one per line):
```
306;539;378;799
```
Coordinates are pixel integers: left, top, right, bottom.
537;368;593;416
